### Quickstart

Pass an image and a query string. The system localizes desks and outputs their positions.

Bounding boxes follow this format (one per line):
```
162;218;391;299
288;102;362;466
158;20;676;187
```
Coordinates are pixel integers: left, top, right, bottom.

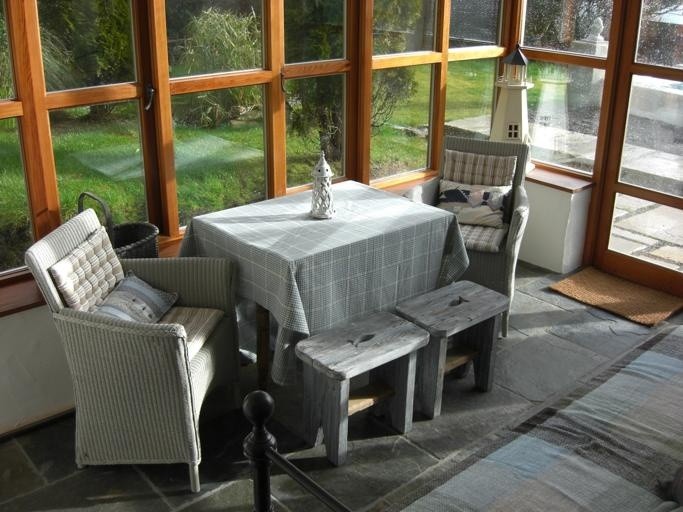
178;180;470;439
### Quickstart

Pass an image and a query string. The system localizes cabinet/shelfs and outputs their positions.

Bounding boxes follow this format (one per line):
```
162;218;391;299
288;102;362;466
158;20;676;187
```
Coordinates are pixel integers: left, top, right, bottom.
518;168;596;274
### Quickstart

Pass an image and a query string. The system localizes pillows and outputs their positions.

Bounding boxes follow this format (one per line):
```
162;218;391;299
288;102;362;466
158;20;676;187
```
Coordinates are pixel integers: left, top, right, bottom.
437;179;512;229
93;269;177;324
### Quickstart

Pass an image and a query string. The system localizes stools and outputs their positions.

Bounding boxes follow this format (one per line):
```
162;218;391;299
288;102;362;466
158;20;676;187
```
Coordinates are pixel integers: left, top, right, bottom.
395;280;510;419
295;311;430;466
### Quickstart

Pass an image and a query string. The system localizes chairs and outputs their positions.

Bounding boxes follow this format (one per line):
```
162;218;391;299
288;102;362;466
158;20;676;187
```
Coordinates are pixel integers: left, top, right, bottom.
24;206;242;492
403;136;530;337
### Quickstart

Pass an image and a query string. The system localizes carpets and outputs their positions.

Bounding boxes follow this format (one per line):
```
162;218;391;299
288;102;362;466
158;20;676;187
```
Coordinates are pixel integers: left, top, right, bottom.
359;314;683;512
547;266;683;327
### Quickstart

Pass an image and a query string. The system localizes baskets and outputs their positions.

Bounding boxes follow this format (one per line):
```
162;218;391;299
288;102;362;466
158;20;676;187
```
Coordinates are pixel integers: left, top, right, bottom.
77;191;159;258
